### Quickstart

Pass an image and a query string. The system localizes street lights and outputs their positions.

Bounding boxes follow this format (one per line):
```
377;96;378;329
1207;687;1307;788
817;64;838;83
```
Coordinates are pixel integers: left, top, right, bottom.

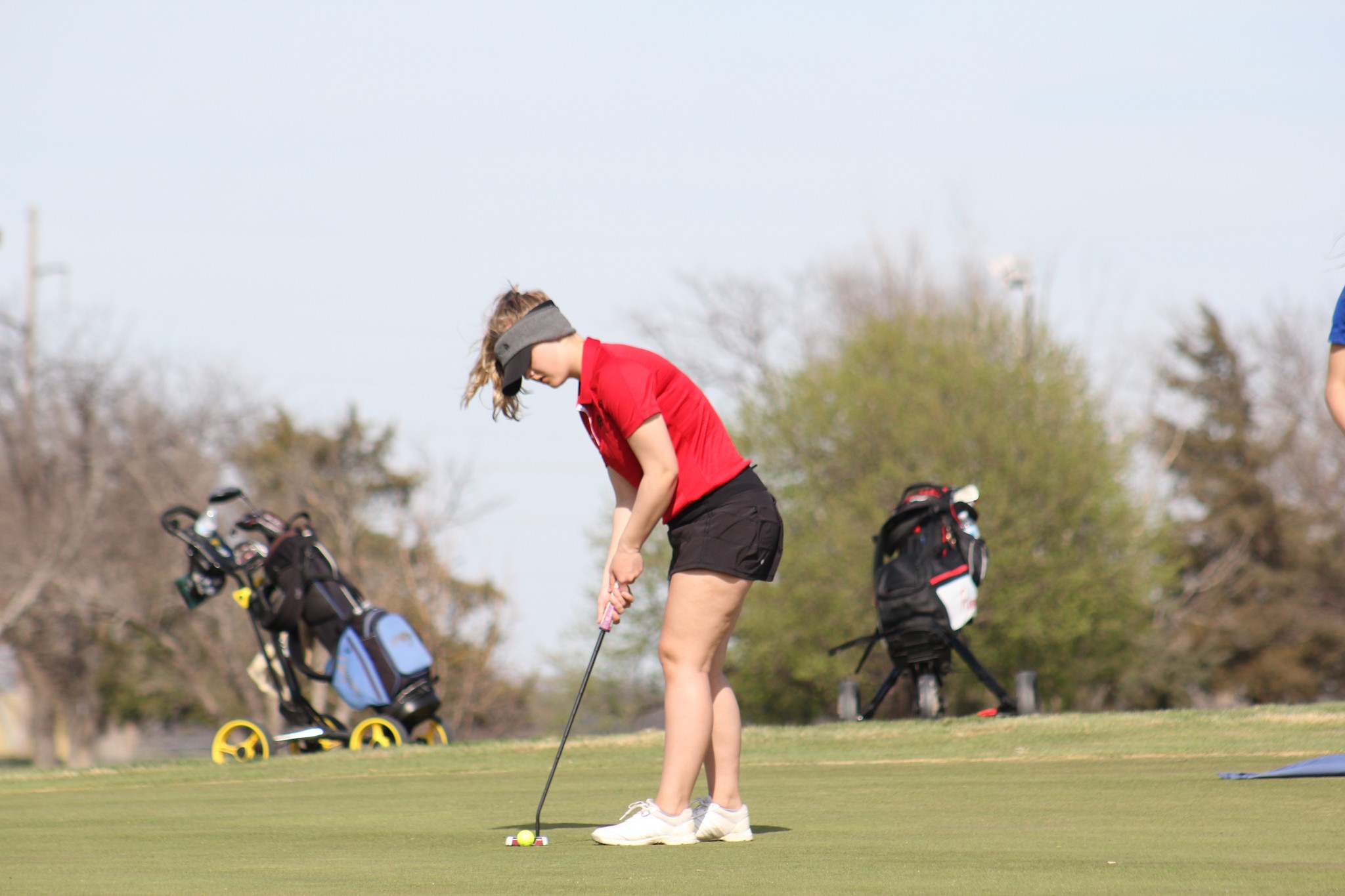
993;255;1035;359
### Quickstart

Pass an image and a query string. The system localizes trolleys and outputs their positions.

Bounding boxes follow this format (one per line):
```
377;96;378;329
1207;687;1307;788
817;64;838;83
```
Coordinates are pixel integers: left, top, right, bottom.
158;500;455;766
825;481;1038;721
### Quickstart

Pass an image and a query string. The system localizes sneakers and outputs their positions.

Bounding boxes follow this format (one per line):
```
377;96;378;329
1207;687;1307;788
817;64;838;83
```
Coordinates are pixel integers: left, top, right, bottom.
689;796;753;842
591;799;696;846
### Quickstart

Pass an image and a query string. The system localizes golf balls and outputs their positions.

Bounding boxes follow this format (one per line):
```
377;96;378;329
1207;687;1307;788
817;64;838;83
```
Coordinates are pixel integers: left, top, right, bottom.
515;829;535;847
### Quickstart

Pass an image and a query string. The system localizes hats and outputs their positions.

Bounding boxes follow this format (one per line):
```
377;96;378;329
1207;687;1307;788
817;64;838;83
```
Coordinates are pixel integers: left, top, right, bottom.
500;300;555;396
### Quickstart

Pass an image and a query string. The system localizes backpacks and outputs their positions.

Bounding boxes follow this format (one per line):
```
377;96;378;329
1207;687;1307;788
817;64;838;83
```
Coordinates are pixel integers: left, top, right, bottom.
875;555;948;671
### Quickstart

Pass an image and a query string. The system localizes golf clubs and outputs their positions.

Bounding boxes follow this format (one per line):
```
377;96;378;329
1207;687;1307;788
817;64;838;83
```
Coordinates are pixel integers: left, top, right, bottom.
505;580;620;847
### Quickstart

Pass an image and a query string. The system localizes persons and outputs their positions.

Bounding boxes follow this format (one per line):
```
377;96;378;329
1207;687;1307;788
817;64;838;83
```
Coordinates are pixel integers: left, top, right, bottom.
1324;286;1345;433
457;286;785;845
